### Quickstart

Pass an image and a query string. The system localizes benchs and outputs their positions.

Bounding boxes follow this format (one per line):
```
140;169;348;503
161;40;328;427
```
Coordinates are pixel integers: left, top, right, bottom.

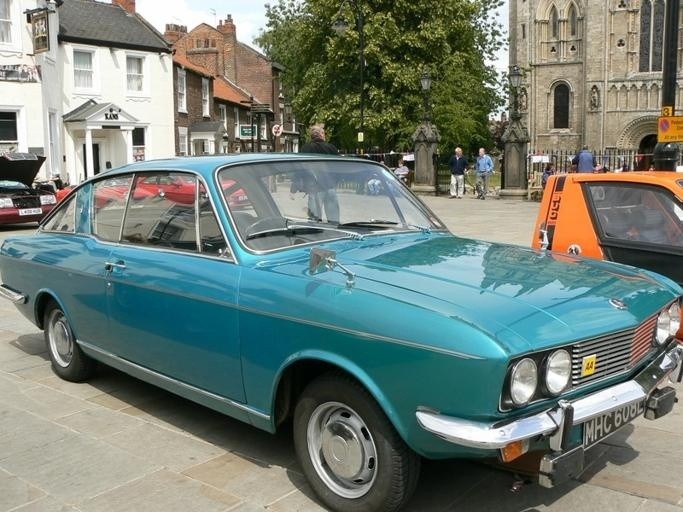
357;167;412;197
528;171;569;201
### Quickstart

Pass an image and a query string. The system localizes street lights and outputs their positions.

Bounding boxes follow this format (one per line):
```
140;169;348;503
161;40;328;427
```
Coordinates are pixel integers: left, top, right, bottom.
508;66;522;110
330;1;364;153
419;68;432;111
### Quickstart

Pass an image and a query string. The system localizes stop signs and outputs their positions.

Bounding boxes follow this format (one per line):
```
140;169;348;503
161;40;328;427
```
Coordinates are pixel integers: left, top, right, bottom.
661;120;669;131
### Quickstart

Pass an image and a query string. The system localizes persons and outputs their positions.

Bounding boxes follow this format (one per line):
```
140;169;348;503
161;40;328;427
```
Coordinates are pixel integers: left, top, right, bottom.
446;147;470;200
540;163;556;191
567;145;655;173
473;147;495;201
392;158;410;198
366;160;386;196
287;125;344;228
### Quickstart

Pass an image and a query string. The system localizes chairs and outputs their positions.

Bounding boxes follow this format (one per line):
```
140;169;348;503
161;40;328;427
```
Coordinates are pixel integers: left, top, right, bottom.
600;213;643;241
632;208;672;245
